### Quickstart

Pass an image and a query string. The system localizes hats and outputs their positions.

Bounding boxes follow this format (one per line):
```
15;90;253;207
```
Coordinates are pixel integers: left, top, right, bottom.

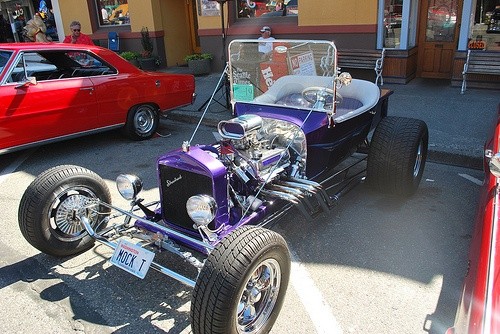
259;26;272;32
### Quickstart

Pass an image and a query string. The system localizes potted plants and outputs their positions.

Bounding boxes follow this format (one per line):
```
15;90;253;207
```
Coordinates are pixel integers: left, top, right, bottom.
185;53;214;77
119;51;159;72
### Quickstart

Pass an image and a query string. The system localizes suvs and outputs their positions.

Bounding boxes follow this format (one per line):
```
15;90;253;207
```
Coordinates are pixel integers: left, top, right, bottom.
16;36;430;334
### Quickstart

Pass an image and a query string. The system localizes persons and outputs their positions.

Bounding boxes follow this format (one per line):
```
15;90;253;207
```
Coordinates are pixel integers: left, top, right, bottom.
63;20;95;66
258;26;275;53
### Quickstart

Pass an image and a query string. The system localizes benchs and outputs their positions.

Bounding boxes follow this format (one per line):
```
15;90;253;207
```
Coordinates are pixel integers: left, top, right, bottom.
286;48;386;86
251;75;380;171
460;49;500;95
53;68;111;78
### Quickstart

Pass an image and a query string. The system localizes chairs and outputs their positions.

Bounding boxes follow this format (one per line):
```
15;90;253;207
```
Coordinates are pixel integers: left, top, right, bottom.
13;71;39;82
46;72;72;80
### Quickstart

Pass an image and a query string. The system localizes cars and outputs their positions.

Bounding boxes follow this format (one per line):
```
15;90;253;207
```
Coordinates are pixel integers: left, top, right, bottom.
0;40;198;155
443;105;500;334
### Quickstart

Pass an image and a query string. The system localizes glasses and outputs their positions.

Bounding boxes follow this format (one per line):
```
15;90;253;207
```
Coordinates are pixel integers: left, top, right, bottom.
72;29;80;32
